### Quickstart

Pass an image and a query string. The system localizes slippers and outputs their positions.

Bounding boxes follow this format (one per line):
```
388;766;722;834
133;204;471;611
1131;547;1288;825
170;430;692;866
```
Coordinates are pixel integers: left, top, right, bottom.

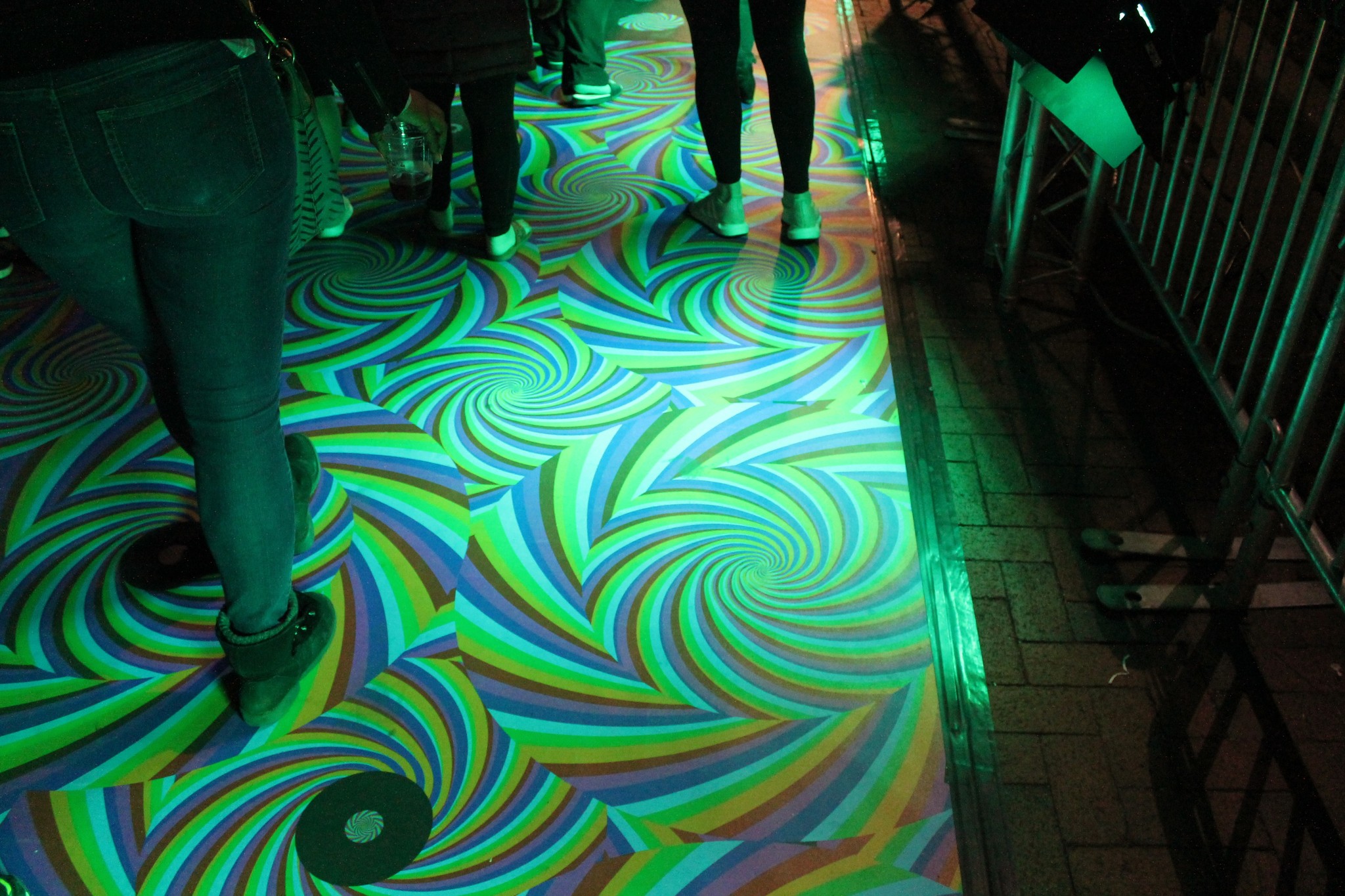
572;79;624;106
488;218;533;262
431;196;455;236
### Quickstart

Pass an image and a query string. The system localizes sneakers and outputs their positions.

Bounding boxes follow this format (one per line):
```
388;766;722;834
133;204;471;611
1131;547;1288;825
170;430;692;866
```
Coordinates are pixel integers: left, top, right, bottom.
781;197;822;240
690;191;749;237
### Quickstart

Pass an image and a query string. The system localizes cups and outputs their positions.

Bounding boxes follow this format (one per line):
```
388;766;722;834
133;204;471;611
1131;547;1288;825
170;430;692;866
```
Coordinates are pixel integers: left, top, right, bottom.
376;120;433;202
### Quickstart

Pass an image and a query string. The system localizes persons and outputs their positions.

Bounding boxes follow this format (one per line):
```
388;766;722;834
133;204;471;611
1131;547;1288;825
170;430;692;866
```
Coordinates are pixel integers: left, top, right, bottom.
499;2;627;111
674;0;830;246
317;0;537;270
0;0;457;727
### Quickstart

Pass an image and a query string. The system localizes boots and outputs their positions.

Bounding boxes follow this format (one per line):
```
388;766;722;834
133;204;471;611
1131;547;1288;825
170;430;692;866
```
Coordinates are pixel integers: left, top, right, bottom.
278;431;322;557
214;586;338;728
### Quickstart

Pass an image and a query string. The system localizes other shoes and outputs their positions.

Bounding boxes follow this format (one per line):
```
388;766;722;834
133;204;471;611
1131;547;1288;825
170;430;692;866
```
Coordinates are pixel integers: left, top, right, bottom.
319;194;354;238
736;64;755;104
